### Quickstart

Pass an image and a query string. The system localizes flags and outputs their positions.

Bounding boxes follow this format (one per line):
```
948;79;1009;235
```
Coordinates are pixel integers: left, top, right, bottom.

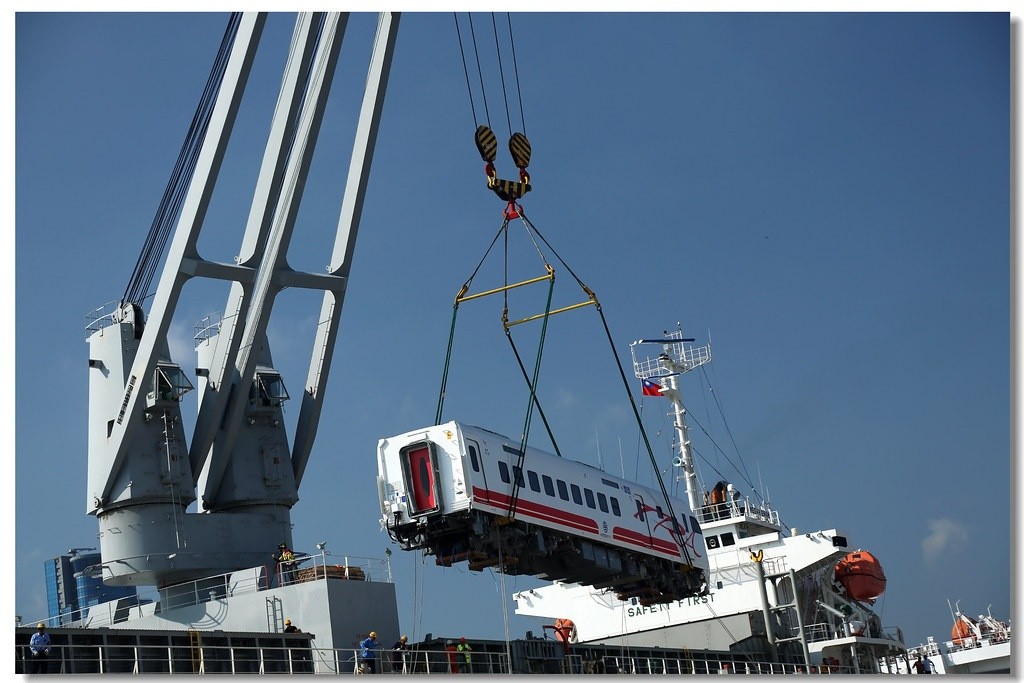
642;379;667;396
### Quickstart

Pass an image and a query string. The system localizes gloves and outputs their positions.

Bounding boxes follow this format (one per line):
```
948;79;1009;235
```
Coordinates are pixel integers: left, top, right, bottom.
365;648;369;651
45;648;49;655
34;651;38;656
396;648;401;650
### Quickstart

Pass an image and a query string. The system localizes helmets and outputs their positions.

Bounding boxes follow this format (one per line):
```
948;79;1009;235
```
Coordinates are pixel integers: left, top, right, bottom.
459;637;465;642
369;631;377;639
285;619;291;625
37;622;45;628
401;635;407;642
279;543;287;549
446;640;453;644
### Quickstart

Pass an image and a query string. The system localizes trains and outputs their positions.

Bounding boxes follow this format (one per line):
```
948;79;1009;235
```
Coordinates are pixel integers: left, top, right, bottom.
376;420;712;605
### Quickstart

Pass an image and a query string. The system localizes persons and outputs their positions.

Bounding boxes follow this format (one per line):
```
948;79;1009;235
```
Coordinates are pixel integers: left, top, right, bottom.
811;663;818;674
271;543;299;585
712;481;729;516
360;631;381;674
283;620;297;633
391;636;409;674
30;622;51;674
456;637;472;674
444;639;458;674
820;658;832;674
724;663;734;674
912;655;935;674
793;667;803;674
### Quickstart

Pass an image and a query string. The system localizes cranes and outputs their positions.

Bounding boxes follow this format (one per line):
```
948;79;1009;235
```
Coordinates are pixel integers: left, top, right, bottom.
87;12;536;609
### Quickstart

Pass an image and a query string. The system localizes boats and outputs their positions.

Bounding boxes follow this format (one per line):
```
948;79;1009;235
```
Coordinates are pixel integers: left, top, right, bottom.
15;321;942;675
877;596;1010;675
